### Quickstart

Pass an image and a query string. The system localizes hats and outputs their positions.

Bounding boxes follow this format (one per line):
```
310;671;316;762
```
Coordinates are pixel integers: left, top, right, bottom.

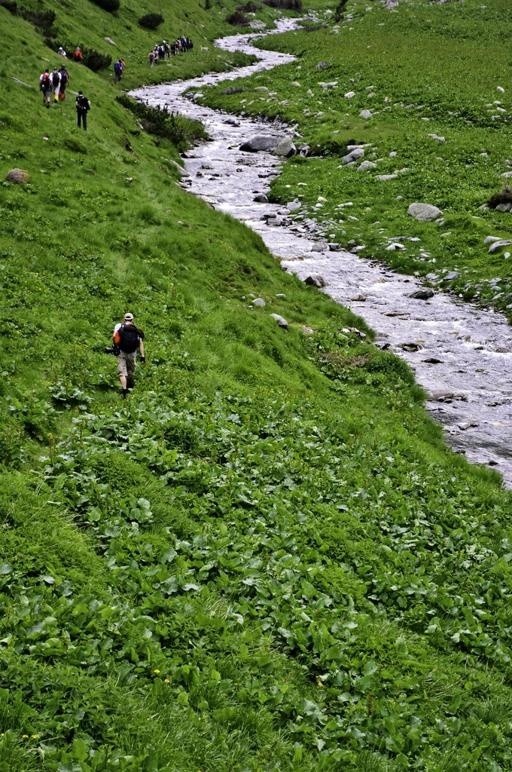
124;312;135;321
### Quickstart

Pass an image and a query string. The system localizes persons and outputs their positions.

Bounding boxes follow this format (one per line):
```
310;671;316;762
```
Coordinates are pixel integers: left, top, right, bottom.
39;64;69;108
73;46;84;64
112;312;146;398
114;58;126;81
148;34;194;68
58;47;67;58
75;90;91;130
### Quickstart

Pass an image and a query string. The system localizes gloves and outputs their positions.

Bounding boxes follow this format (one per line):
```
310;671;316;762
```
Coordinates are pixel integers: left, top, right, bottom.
138;356;146;363
